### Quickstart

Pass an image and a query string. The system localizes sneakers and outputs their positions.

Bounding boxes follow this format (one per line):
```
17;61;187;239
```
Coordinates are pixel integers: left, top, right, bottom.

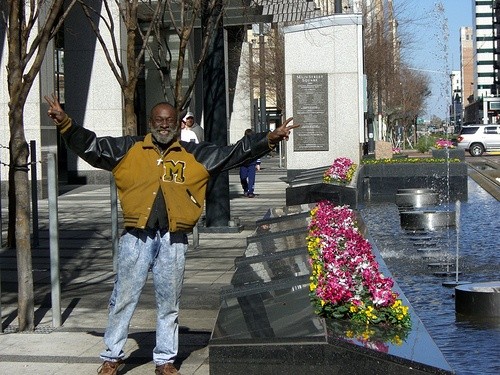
155;363;181;375
98;360;126;375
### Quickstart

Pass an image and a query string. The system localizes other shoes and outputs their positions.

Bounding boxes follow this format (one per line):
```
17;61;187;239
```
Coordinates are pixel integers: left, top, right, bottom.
243;184;248;196
249;193;253;198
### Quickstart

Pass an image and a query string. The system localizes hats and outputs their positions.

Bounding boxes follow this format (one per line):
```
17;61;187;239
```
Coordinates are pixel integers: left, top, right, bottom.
185;111;194;119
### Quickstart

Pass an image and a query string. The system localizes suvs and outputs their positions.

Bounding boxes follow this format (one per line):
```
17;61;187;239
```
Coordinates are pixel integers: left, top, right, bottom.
455;124;500;157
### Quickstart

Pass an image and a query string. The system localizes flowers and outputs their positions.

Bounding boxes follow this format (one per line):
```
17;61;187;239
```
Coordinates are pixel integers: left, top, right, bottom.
305;199;412;333
323;157;357;185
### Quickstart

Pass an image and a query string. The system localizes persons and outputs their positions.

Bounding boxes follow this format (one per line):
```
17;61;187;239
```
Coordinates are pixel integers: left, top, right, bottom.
240;129;261;197
180;117;200;144
43;94;302;375
184;111;204;143
